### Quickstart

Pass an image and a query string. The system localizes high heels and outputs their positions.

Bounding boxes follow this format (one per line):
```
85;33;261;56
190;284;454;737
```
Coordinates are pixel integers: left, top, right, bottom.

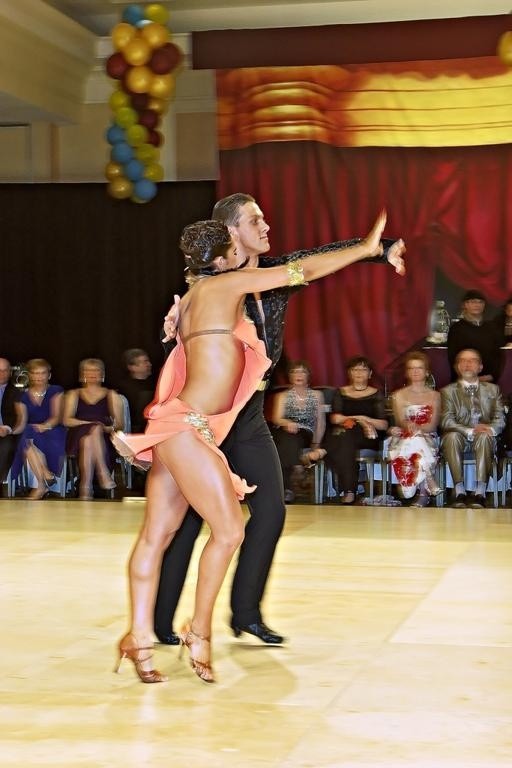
152;624;180;645
231;620;283;645
115;632;167;683
178;619;216;682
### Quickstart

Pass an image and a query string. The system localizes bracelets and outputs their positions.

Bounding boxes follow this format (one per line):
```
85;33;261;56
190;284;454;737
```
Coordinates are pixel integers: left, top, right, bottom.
46;423;52;431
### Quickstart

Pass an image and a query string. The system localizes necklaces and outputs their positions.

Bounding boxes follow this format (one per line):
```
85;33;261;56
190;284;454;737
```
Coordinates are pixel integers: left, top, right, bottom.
33;389;47;397
297;391;306;401
353;385;368;391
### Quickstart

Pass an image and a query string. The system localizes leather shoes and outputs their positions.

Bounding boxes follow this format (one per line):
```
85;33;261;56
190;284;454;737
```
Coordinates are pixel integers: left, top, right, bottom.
472;494;485;508
455;493;467;508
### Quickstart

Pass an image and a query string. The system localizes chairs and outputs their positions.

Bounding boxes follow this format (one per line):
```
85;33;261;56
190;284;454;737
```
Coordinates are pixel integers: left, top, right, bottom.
3;385;512;507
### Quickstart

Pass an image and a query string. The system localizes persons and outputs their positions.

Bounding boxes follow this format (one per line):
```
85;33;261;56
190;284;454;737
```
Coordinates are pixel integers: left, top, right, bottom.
493;301;512;346
439;349;505;509
388;351;444;508
270;360;327;502
447;289;502;385
111;210;388;683
0;347;158;498
322;356;390;505
151;191;406;644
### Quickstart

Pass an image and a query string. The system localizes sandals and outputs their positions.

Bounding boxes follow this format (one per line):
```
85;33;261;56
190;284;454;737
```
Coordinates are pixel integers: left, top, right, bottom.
409;494;431;507
431;486;444;496
